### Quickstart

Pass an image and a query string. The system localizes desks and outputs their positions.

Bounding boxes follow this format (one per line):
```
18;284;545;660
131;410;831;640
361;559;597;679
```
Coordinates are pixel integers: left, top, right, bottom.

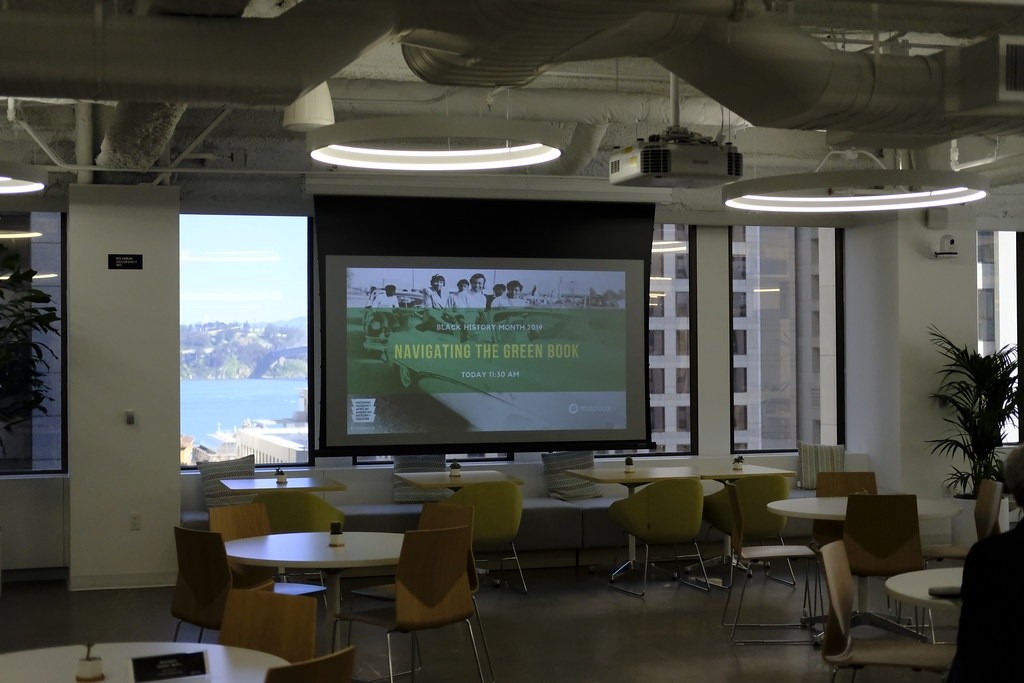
0;642;292;683
565;463;797;587
766;496;963;645
395;470;525;592
884;566;965;644
225;532;404;656
219;477;347;583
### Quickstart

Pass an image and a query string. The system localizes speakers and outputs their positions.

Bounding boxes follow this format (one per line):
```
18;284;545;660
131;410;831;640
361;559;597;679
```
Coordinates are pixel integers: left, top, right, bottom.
927;206;949;230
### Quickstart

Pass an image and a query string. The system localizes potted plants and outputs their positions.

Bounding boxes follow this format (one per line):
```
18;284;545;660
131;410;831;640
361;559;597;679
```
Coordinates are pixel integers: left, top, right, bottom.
927;322;1019;547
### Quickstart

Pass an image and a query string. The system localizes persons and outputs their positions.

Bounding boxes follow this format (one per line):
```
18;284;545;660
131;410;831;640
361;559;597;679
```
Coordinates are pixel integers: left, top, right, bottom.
948;444;1024;683
367;270;549;342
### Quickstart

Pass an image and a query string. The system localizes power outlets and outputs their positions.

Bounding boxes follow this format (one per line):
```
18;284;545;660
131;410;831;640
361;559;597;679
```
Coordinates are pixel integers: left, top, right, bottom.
128;511;141;531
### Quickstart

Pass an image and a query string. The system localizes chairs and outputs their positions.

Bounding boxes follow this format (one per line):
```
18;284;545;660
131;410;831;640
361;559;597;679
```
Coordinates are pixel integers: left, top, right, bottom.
170;472;1005;683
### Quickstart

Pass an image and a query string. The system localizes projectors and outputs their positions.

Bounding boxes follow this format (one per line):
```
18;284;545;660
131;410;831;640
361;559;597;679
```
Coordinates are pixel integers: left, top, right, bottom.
608;142;743;190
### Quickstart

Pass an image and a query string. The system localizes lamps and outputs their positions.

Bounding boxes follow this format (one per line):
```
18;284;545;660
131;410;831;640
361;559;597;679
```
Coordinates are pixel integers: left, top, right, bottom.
0;161;48;195
721;148;990;212
282;80;334;133
308;89;564;171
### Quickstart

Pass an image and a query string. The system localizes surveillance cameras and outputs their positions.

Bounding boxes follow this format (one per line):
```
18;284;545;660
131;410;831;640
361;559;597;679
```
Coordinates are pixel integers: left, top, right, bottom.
939;235;959;256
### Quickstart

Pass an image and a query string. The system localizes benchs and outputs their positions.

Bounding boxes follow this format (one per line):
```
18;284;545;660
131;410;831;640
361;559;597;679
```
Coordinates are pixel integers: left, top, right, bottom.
179;452;881;569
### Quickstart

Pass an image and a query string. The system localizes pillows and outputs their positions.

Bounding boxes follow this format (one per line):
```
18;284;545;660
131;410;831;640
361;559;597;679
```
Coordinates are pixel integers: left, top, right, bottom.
795;440;846;490
540;450;605;501
392;455;453;504
196;454;257;512
